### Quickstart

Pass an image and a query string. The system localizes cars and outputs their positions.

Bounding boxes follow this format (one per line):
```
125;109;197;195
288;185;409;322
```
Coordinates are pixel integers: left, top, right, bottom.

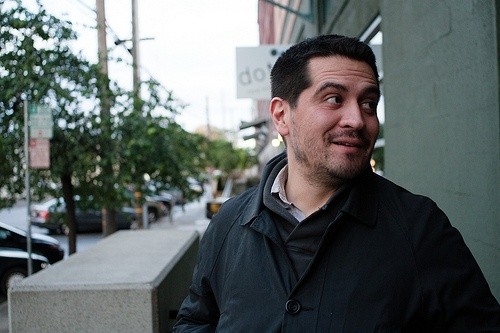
30;176;203;235
0;222;63;302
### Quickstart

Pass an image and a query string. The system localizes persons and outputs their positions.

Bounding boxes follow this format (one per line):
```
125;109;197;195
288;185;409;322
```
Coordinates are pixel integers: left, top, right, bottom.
172;35;500;333
